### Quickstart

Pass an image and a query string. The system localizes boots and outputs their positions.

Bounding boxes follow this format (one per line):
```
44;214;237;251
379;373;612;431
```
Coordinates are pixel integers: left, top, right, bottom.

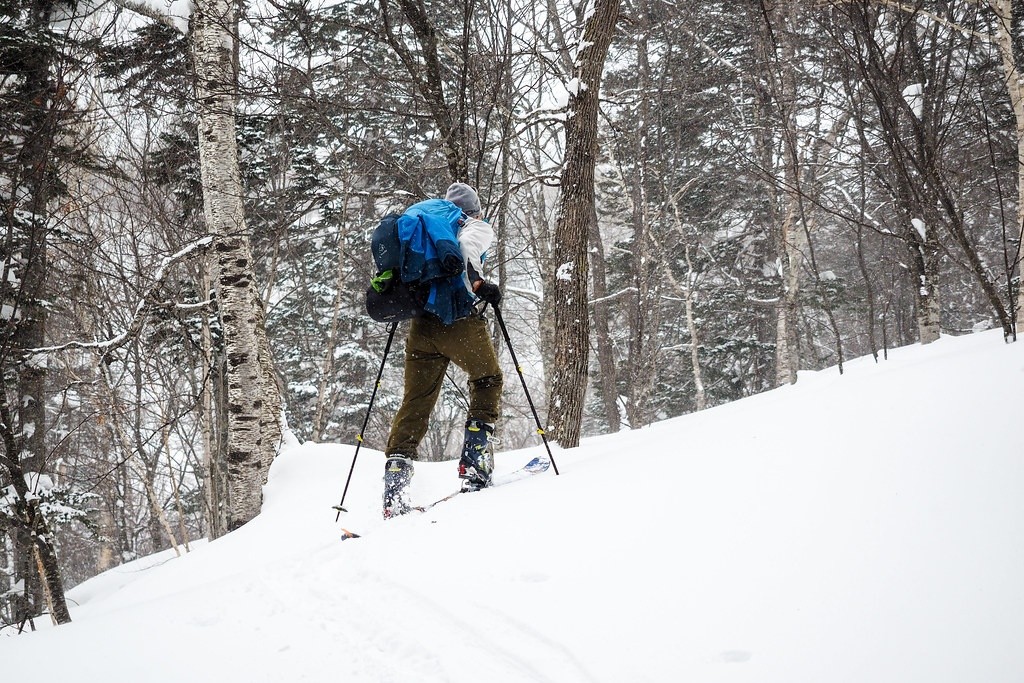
382;459;415;519
457;418;494;487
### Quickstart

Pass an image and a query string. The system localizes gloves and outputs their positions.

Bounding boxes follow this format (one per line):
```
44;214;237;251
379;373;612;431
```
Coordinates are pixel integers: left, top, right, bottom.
473;280;502;309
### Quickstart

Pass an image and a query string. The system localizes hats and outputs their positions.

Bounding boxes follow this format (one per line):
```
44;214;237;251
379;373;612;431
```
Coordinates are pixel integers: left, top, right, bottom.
445;183;481;217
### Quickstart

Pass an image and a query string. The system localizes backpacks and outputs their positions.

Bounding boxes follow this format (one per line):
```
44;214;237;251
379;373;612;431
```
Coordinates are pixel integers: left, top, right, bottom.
366;213;430;322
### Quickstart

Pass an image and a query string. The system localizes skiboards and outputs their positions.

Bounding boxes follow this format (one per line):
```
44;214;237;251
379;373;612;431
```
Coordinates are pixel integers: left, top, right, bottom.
340;454;552;541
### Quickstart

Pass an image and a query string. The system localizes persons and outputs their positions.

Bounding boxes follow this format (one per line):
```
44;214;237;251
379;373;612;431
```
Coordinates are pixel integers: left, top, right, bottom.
381;183;504;520
